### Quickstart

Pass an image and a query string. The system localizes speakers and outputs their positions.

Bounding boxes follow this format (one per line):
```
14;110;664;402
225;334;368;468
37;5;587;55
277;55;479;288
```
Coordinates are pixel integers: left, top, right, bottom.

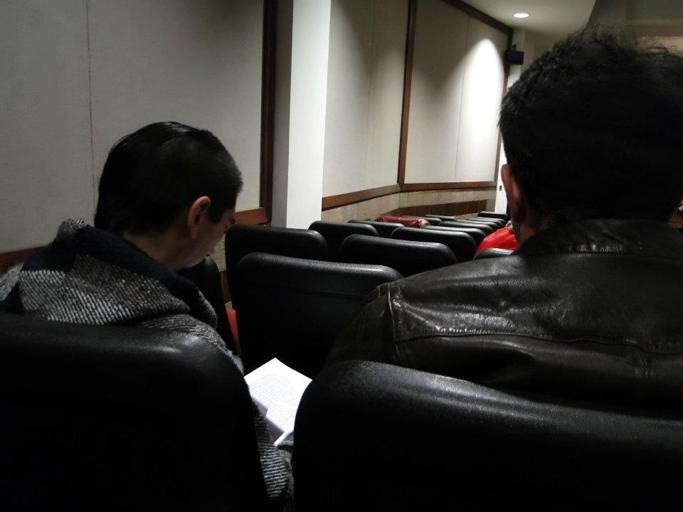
505;48;524;67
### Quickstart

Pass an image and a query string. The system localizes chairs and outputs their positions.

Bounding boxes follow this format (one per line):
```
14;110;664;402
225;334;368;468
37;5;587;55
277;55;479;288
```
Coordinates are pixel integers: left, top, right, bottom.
0;210;681;509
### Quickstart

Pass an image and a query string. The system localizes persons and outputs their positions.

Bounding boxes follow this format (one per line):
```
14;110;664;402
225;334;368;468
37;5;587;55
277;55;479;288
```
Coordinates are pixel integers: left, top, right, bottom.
0;119;299;505
327;26;682;407
